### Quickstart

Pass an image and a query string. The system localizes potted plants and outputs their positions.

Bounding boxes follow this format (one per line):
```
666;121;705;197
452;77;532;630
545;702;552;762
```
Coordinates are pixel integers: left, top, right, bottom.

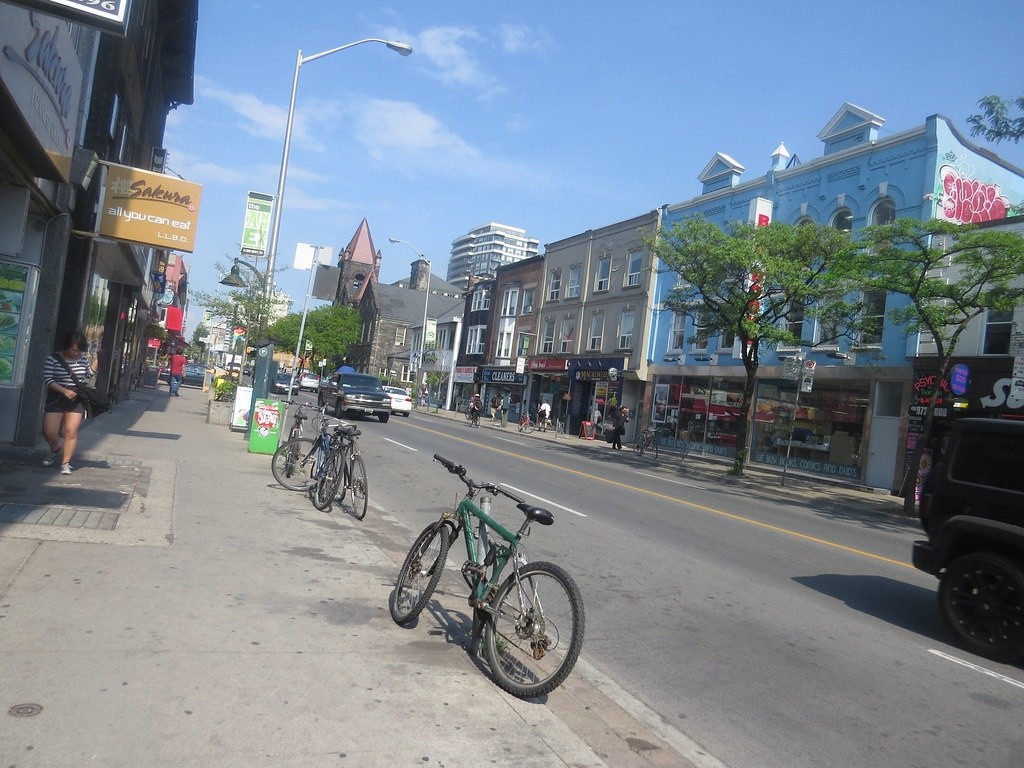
207;374;237;425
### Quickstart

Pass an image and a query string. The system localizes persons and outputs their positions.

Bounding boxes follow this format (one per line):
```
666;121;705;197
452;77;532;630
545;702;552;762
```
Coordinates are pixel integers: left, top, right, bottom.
490;393;500;422
467;393;482;420
42;333;98;475
421;384;427;406
169;348;186;396
612;406;629;450
405;385;412;396
537;399;551;432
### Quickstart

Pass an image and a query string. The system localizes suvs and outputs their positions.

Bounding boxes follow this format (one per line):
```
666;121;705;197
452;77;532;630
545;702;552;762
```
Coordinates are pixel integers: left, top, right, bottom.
910;414;1024;660
317;371;392;423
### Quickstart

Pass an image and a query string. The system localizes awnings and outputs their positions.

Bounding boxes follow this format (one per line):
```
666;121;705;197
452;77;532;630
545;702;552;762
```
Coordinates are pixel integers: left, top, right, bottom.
166;305;182;331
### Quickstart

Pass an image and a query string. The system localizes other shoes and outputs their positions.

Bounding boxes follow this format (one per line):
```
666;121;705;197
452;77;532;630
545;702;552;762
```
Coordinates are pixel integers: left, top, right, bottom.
173;389;179;396
170;393;174;396
612;447;622;450
538;429;546;432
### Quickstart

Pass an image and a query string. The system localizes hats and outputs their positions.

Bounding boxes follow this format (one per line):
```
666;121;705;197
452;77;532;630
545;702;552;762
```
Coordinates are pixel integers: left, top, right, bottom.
473;394;480;396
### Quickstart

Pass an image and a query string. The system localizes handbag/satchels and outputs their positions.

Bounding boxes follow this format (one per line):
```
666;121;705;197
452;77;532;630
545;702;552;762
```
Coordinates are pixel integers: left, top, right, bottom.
538;410;546;417
81;385;110;419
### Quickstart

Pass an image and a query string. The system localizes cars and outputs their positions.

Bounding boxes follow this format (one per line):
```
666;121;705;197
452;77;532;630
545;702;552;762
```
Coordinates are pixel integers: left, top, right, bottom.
145;356;255;387
380;385;413;417
270;373;301;396
298;371;320;393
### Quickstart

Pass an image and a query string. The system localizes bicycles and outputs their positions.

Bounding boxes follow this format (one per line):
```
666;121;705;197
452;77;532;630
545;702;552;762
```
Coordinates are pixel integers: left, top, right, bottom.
280;399;321;478
518;409;536;434
464;401;481;429
271;400;369;520
633;427;662;460
390;452;586;700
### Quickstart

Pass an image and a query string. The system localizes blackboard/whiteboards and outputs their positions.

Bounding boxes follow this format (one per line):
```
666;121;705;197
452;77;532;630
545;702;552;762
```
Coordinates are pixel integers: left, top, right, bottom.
579;421;594;440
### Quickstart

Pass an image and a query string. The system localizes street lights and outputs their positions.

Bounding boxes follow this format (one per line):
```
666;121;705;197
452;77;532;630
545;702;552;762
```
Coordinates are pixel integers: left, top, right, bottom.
260;37;414;336
388;236;432;407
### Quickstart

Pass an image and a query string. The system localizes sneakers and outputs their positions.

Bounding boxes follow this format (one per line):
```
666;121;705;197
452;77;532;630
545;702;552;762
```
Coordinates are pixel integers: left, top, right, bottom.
42;453;57;466
60;463;73;475
